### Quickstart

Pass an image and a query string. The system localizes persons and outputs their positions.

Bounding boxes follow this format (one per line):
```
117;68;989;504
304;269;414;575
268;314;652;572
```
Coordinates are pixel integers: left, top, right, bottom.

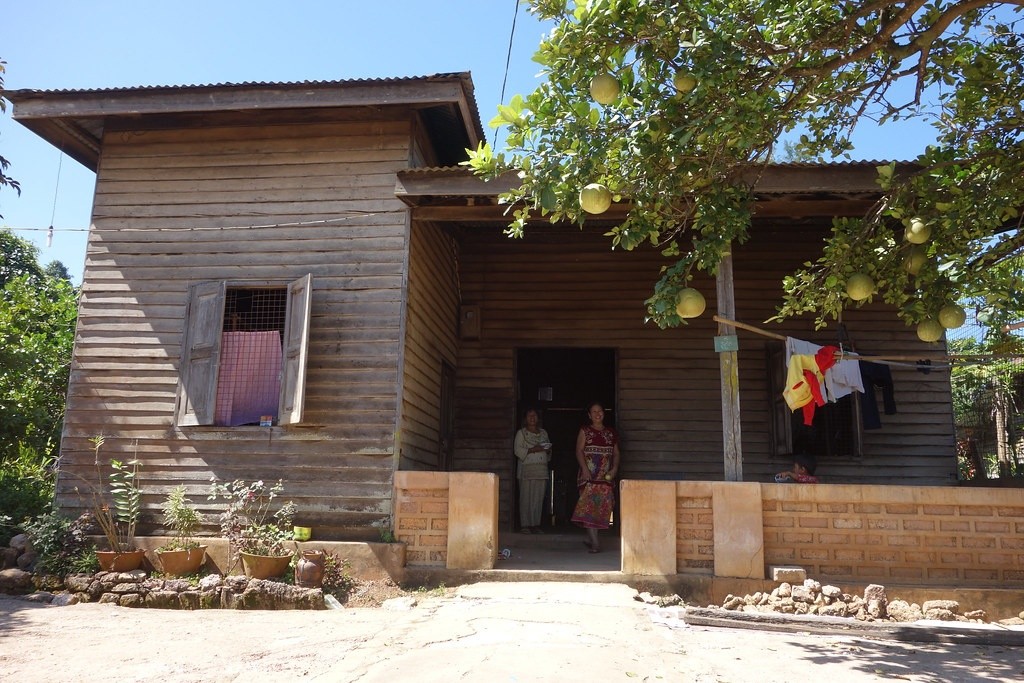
778;453;819;484
571;400;622;554
514;407;552;534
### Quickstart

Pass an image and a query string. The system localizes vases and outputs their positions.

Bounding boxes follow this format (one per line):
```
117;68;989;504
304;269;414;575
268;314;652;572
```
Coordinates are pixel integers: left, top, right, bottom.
294;525;311;541
296;549;325;587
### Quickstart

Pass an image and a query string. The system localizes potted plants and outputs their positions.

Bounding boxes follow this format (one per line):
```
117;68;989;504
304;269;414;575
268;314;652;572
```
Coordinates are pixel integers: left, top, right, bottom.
153;485;207;578
207;476;294;580
57;434;148;571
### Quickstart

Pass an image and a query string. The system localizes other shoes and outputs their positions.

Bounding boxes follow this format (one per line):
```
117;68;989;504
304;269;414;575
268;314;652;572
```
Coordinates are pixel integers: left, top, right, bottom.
521;528;531;534
582;540;591;547
532;528;544;533
590;548;601;554
